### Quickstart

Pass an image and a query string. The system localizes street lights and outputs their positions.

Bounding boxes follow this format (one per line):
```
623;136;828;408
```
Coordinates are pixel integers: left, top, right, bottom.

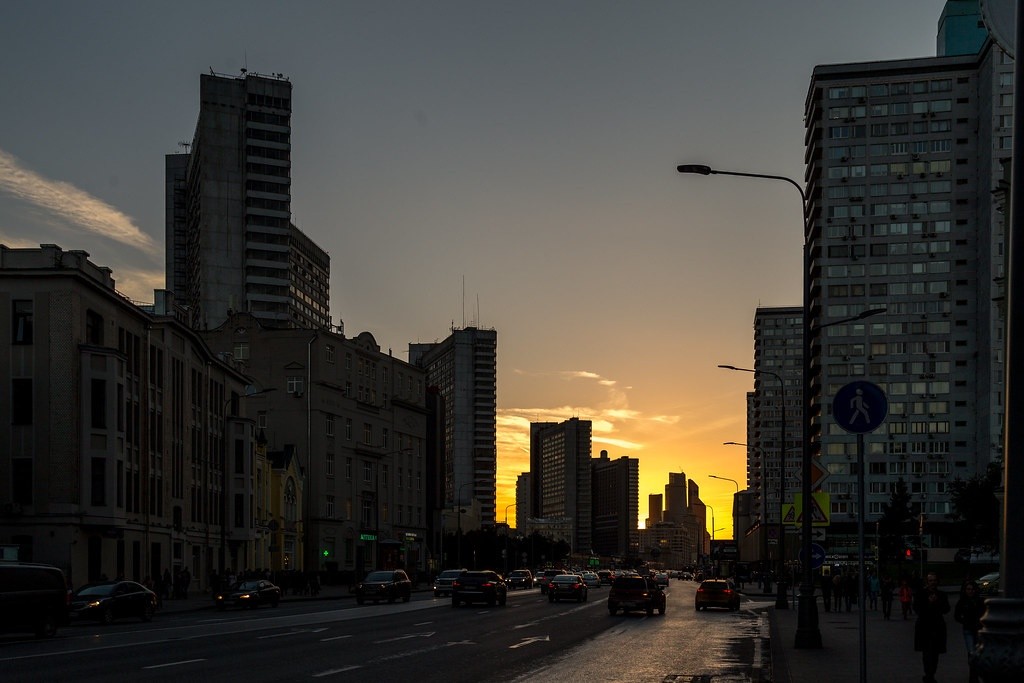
376;447;415;550
717;363;789;601
505;501;526;524
792;308;888;653
721;442;771;594
709;475;740;559
457;478;488;531
217;384;279;580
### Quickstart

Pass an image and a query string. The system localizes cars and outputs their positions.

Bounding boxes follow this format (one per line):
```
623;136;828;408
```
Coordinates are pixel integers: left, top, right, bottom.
450;570;508;609
214;582;281;611
694;579;740;612
70;581;159;623
547;575;589;601
505;568;693;594
355;568;412;603
433;568;469;599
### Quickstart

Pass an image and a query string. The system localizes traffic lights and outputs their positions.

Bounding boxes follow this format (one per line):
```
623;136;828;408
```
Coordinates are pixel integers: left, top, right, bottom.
905;547;915;559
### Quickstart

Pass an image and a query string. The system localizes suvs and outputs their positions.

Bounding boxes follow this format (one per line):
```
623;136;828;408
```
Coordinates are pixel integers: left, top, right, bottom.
0;561;72;641
608;575;667;617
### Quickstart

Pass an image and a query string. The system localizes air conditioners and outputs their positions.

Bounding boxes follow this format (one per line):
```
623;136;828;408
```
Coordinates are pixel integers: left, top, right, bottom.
846;455;852;459
939;292;948;298
927;435;935;438
845;495;851;499
826;193;920;222
889;435;895;439
851;252;936;261
842;354;874;360
937;454;944;459
929;353;937;359
843;96;936;123
920;312;929;319
900;374;938;418
927;454;936;459
841;231;938;241
920;494;926;498
900;455;908;459
840;153;943;182
846;514;855;518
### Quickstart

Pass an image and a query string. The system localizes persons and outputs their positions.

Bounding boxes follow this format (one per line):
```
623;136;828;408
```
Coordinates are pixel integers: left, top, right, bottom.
309;571;318;596
912;572;950;683
172;566;190;601
899;580;912;620
821;569;859;613
955;580;984;655
209;570;217;590
280;570;307;598
881;576;896;621
246;568;274;584
151;568;163;604
867;570;879;611
163;568;171;600
